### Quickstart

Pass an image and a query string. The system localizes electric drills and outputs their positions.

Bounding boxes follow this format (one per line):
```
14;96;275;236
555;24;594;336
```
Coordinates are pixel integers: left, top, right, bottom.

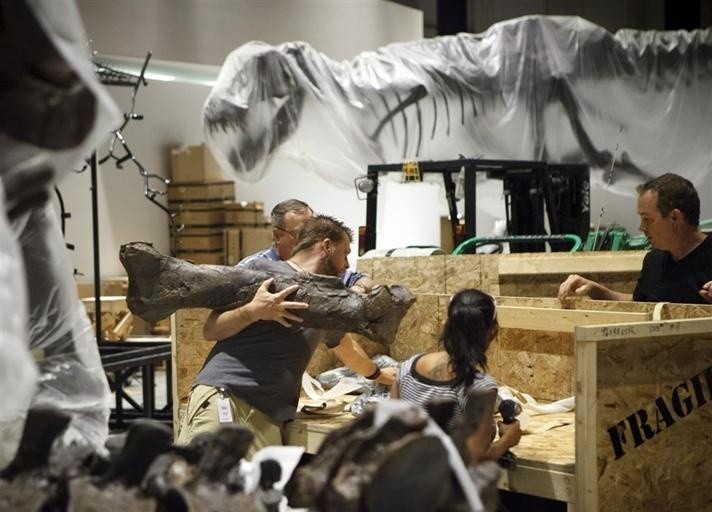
496;399;524;471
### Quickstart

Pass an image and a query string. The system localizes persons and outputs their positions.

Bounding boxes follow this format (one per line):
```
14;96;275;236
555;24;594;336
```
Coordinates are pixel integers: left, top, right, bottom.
172;214;399;464
558;171;712;304
203;196;378;344
387;286;523;472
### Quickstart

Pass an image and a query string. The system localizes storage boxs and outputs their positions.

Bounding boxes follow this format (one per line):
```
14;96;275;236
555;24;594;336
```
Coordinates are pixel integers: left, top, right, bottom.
164;140;274;267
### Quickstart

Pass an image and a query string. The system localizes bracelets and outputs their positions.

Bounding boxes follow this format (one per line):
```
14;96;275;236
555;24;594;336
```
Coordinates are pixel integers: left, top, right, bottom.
363;365;382;382
355;282;370;292
